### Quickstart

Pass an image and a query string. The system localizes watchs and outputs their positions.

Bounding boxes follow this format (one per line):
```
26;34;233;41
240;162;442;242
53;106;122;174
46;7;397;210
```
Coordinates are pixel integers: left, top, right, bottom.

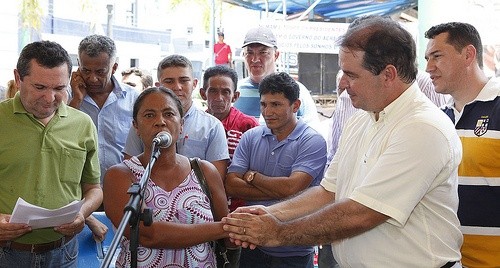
247;171;256;183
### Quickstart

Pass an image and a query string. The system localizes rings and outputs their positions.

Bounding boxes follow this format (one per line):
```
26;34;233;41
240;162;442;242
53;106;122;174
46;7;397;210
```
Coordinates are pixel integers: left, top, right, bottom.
243;227;245;235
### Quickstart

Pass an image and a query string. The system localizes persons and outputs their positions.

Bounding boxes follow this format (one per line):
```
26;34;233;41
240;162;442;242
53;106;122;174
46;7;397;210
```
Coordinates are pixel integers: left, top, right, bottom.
122;69;153;92
222;18;463;268
224;72;327;268
200;67;260;213
103;85;233;268
65;34;141;187
424;22;500;268
0;41;103;268
215;32;232;68
316;13;452;268
121;55;230;181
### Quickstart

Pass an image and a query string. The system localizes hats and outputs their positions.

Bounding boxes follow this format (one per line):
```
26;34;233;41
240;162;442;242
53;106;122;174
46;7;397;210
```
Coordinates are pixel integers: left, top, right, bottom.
241;28;278;49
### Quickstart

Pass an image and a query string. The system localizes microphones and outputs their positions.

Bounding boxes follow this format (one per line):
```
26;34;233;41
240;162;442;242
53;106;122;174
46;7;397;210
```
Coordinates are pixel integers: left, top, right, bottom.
152;131;173;149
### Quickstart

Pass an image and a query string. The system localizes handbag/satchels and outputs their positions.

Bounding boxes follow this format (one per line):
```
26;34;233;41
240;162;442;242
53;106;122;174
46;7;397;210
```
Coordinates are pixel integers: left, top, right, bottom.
215;238;232;268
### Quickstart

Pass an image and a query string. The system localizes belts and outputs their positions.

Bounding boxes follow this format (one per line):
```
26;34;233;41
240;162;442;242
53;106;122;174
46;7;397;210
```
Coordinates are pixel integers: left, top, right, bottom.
0;234;75;253
441;261;457;268
